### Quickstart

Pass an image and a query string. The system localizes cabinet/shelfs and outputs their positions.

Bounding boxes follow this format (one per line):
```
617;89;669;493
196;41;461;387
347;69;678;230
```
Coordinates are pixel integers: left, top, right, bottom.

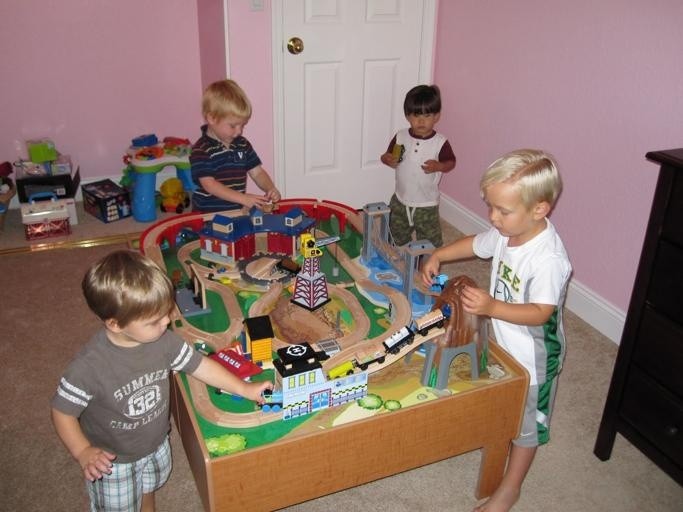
593;146;682;490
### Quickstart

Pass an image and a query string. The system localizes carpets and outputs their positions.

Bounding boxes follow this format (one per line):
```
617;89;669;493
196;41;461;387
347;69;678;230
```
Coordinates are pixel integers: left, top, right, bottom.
1;213;683;510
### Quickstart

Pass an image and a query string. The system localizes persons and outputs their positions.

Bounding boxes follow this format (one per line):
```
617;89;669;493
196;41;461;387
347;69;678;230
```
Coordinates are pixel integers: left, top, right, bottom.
417;148;573;511
188;78;282;214
49;248;277;512
379;84;457;273
